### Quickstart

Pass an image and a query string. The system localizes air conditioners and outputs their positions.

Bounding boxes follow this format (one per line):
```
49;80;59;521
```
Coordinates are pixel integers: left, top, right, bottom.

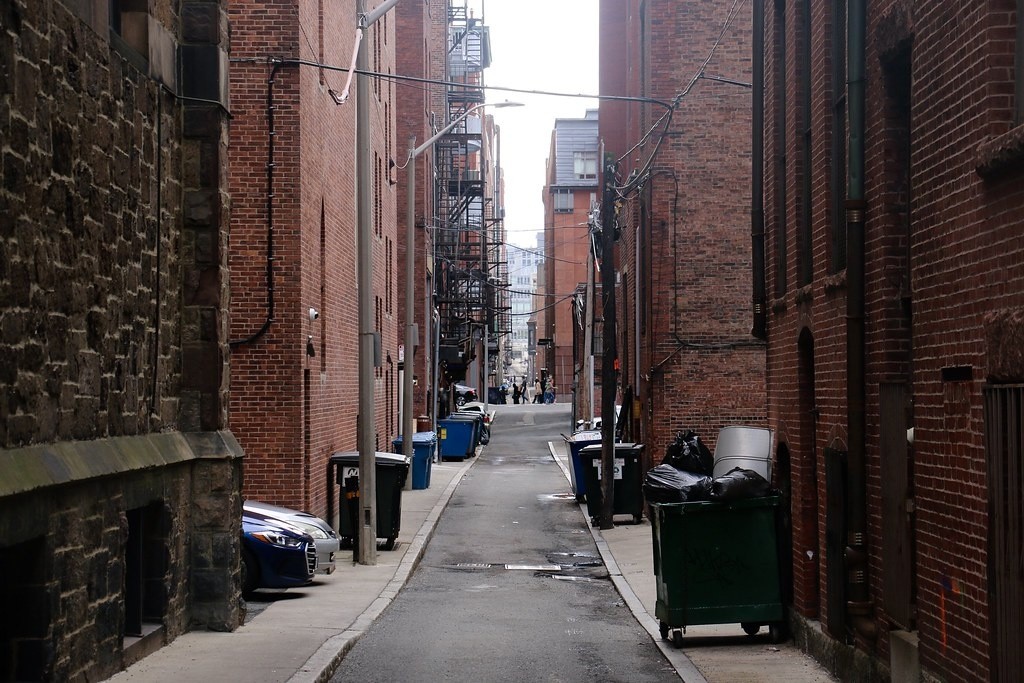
462;170;480;188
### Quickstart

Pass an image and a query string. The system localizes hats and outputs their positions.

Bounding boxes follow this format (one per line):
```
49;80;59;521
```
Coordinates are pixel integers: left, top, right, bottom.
502;386;506;389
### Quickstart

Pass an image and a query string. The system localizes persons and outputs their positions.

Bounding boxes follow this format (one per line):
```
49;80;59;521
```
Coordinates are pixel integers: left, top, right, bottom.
500;385;509;404
512;383;521;404
541;374;558;404
532;379;542;404
520;376;528;404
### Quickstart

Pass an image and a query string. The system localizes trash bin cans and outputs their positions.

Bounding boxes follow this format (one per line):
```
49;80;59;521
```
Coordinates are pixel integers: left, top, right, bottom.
563;437;620;503
489;386;499;404
578;443;647;525
393;431;437;490
437;411;485;462
646;496;792;648
329;450;410;549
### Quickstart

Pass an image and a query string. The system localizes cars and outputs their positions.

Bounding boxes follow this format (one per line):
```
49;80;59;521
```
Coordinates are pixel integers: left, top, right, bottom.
454;384;478;407
238;499;340;601
